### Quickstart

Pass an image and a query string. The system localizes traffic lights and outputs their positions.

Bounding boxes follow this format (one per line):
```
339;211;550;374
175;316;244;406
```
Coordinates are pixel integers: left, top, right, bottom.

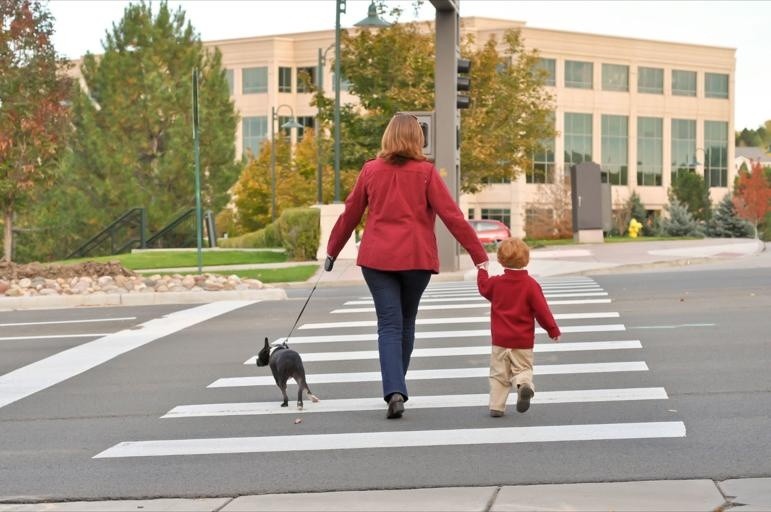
453;51;470;109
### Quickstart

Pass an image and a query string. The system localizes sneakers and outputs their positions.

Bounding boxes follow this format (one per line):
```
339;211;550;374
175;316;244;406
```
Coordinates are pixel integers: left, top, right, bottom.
517;383;530;412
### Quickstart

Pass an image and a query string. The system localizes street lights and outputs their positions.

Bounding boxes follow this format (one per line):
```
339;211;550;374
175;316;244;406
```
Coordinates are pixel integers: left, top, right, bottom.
271;102;305;226
689;147;714;241
316;38;357;205
333;0;398;208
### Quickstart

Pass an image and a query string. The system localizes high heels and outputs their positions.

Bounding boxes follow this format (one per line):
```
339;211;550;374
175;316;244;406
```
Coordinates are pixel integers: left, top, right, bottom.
388;393;404;417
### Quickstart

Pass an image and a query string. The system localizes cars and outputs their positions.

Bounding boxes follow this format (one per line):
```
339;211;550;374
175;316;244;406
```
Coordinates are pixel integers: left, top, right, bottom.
463;218;512;247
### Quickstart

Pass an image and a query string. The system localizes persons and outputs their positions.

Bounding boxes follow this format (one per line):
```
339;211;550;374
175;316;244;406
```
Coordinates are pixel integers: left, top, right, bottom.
478;237;561;417
327;113;489;420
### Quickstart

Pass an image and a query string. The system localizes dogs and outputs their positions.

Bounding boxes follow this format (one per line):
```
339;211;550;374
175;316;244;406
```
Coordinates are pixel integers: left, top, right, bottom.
256;337;320;410
